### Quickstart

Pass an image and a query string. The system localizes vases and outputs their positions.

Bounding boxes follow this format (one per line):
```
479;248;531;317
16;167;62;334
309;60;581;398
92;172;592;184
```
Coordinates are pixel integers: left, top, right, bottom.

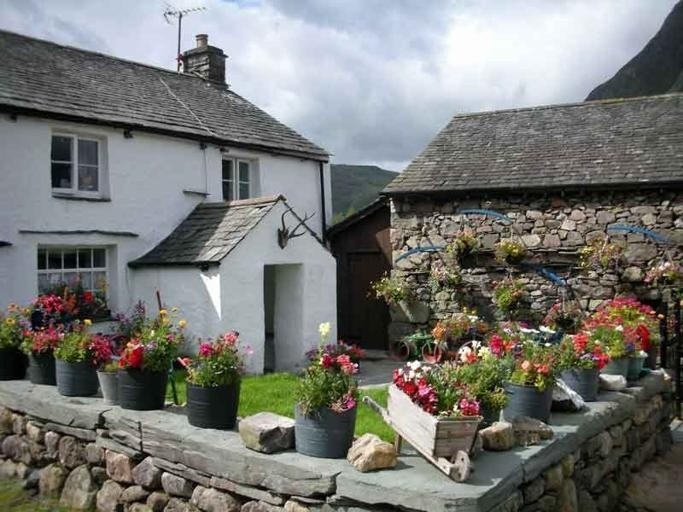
387;384;483;461
185;384;240;430
118;372;168;411
502;381;555;422
294;407;356;459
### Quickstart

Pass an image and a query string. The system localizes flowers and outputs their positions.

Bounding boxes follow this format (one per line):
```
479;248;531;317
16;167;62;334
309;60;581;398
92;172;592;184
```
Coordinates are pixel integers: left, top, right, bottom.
183;330;252;390
0;279;145;404
294;321;366;419
117;304;187;373
